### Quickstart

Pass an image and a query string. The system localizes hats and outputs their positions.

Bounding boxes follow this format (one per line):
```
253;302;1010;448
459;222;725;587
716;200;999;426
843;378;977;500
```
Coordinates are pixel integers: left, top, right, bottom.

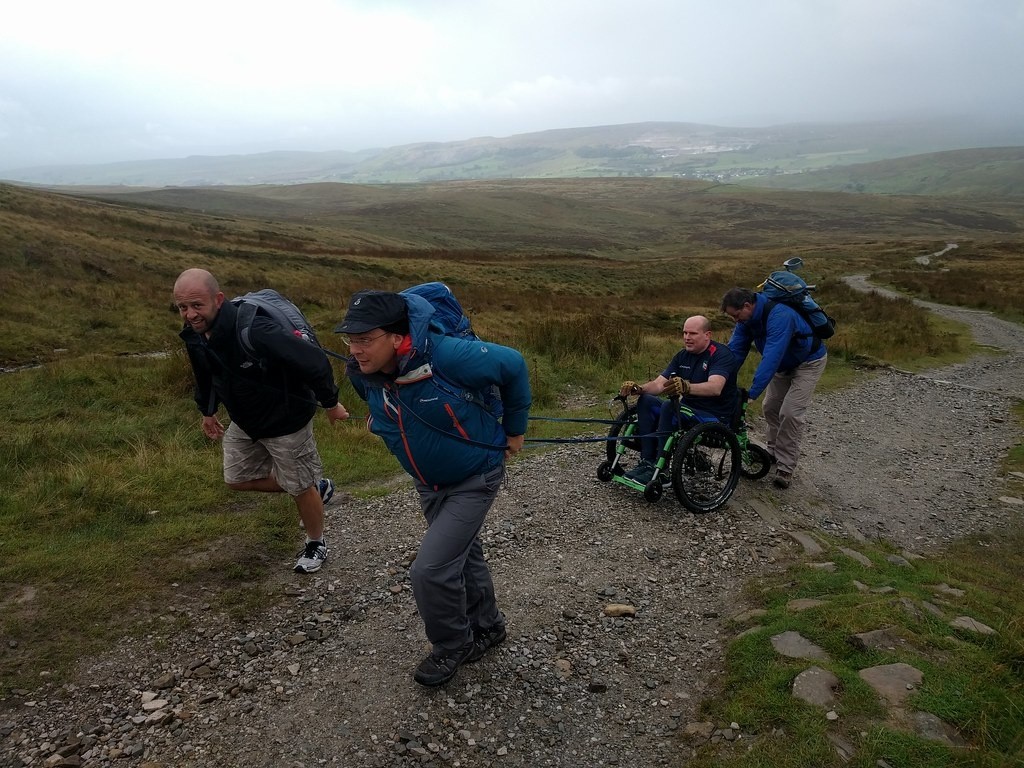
333;291;409;334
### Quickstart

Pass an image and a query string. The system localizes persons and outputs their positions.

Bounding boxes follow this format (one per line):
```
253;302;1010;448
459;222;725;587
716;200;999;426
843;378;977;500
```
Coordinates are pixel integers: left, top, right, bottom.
618;314;739;487
173;268;350;575
722;286;828;488
334;289;531;689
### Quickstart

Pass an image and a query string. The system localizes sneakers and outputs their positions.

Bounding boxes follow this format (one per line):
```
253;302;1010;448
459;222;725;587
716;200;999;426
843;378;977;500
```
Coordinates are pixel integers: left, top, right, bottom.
414;637;475;687
293;537;327;573
773;468;791;488
299;478;334;531
624;458;672;488
747;448;776;464
464;623;506;663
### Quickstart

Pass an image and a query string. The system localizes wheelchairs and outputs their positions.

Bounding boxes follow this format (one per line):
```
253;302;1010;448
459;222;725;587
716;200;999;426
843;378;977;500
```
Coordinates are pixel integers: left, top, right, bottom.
596;363;774;514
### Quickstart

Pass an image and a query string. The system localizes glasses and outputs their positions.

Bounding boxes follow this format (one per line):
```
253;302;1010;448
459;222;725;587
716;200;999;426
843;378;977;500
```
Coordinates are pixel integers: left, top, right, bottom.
341;331;390;348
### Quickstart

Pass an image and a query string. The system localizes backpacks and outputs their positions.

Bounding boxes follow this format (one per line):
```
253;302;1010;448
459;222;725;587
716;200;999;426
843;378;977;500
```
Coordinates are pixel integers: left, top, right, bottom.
756;271;836;354
230;288;325;369
397;283;505;419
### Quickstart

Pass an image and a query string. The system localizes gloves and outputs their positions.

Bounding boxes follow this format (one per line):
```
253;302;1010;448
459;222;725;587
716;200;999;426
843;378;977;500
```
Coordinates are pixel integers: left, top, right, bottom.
617;380;643;398
663;377;690;395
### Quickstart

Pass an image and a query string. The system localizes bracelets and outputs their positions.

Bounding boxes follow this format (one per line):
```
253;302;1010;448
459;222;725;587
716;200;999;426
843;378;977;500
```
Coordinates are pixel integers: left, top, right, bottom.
325;402;339;411
